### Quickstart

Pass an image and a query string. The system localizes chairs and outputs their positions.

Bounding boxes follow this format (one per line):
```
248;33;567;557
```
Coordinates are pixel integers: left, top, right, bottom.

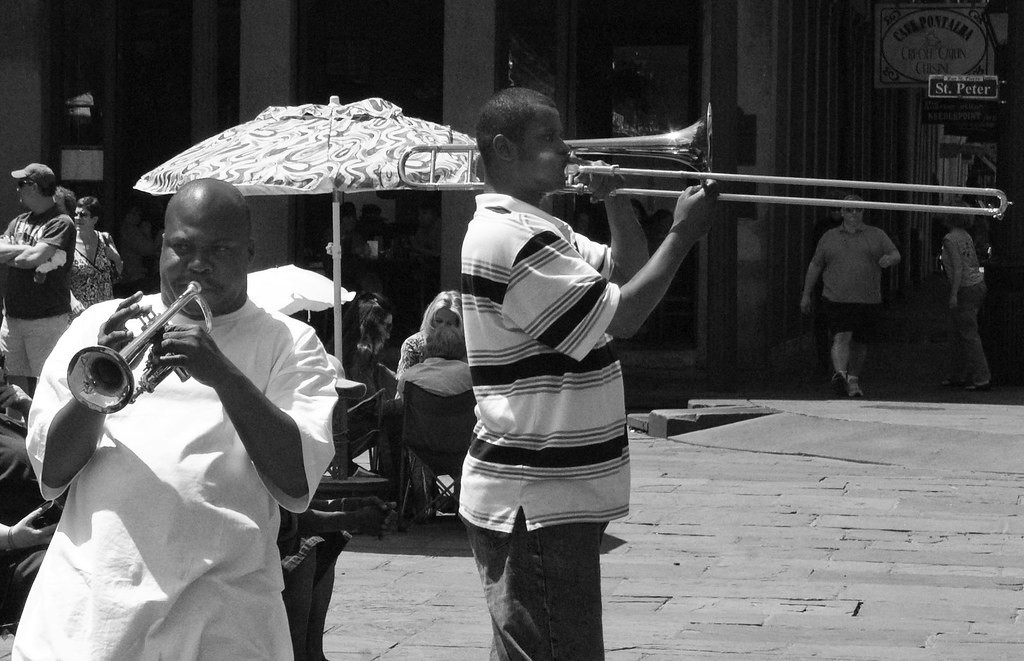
395;379;477;530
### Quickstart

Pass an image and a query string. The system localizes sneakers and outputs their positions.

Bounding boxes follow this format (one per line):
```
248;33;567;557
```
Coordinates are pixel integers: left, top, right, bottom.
831;371;846;394
846;375;863;397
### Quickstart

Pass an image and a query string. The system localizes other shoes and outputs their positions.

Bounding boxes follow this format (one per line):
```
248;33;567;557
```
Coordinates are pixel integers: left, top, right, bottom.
942;375;991;391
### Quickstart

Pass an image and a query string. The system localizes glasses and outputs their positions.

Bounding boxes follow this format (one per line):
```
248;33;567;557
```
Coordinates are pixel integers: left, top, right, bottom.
846;207;863;212
74;212;93;218
379;320;392;332
18;181;34;188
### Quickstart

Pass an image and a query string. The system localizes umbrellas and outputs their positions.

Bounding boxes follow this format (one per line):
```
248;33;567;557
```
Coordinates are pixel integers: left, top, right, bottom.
132;95;489;471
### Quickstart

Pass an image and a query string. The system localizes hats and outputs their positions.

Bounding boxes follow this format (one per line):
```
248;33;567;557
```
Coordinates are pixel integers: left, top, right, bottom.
11;163;55;188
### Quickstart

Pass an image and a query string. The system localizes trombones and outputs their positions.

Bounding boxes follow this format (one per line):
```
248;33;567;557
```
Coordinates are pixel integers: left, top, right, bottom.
399;101;1008;219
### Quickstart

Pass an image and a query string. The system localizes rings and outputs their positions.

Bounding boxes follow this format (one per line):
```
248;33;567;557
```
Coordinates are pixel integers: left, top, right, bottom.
887;260;890;263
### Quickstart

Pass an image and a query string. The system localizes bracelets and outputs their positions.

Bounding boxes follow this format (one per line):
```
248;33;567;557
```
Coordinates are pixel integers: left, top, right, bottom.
6;526;17;551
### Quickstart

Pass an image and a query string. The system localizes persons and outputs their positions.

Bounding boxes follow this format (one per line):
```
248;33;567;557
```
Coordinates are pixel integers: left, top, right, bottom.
303;187;490;522
5;177;344;661
64;194;125;327
0;344;75;629
276;486;399;661
0;161;78;398
933;199;993;392
114;200;164;296
52;185;77;219
452;83;708;661
801;192;903;398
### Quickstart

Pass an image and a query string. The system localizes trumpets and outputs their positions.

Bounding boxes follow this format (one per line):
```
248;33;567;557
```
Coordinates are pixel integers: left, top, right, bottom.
64;282;214;416
